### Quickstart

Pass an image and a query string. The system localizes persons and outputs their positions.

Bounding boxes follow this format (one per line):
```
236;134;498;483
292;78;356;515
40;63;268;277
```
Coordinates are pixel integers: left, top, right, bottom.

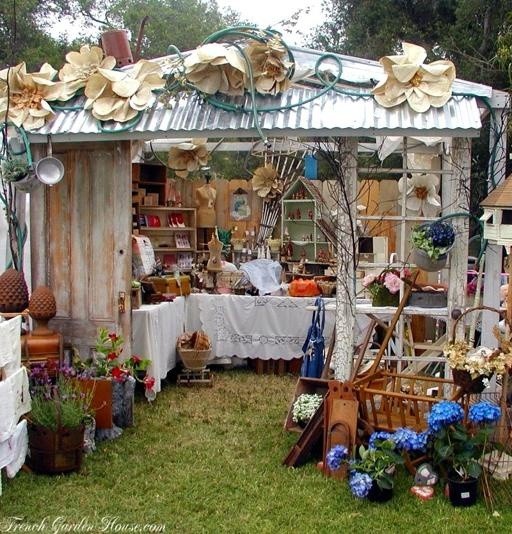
194;184;219;228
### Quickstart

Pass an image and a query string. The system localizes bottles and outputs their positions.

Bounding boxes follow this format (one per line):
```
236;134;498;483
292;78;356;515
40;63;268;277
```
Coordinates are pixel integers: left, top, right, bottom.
281;186;314;260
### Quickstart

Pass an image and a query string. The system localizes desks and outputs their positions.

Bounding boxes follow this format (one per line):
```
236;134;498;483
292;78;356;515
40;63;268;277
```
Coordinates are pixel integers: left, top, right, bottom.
131;292;185;402
185;291;372;376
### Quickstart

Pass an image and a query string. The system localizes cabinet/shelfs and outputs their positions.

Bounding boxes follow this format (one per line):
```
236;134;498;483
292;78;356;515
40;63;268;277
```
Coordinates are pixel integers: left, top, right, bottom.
140;206;198;274
279;176;337;266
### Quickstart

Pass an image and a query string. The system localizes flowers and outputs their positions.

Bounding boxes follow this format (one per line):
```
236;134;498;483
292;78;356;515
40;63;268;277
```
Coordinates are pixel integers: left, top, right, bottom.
23;360;110;431
397;175;443;218
414;401;502;480
412;223;454;258
0;40;456;126
167;136;213;179
325;427;426;497
251;162;285;200
442;341;512;380
70;326;155;389
361;265;412;297
291;392;324;425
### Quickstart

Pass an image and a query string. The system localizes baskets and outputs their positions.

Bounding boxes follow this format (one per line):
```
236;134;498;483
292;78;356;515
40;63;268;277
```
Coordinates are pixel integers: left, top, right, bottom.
179;331;212;369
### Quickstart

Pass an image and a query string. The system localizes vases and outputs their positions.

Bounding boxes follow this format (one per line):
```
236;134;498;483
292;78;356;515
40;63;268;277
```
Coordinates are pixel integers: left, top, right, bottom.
446;477;478;507
111;380;134;428
451;368;493;394
411;236;454;272
366;480;394;504
371;289;400;307
29;424;89;475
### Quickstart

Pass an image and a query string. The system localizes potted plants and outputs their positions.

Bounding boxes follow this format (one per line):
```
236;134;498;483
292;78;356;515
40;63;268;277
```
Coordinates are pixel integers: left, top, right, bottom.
1;160;40;194
